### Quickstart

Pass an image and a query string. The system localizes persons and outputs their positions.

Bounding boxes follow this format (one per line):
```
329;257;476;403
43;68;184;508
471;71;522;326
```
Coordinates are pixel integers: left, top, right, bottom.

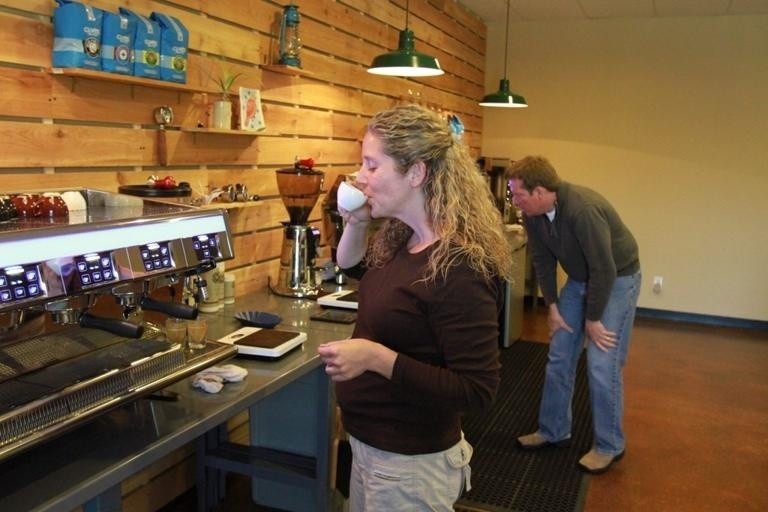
506;154;641;473
319;104;513;512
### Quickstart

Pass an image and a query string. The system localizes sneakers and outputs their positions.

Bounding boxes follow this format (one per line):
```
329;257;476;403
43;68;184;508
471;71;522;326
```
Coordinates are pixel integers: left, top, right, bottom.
579;448;624;474
518;430;571;449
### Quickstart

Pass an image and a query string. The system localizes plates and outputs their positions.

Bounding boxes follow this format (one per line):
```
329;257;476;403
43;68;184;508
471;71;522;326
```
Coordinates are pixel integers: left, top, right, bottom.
232;310;282;327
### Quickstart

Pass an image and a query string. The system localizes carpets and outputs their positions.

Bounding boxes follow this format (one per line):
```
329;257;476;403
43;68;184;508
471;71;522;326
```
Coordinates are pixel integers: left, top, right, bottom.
451;338;597;512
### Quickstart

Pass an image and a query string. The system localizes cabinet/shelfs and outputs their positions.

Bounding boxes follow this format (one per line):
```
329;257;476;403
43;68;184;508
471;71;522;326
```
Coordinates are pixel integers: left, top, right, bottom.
36;65;281;212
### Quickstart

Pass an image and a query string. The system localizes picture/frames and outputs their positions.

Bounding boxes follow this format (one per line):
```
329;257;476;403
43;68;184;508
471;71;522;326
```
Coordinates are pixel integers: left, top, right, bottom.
237;86;266;132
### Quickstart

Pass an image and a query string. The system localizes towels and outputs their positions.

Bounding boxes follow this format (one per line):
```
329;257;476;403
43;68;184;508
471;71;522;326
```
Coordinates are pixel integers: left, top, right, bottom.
191;363;249;394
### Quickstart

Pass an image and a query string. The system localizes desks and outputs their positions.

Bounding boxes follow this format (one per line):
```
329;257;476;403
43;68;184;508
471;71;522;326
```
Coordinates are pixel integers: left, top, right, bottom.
205;232;528;512
0;313;355;512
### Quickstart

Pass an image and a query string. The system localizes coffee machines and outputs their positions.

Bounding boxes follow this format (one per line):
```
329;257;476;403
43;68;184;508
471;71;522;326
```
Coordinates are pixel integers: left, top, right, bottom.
1;184;242;477
324;201;346;285
275;165;326;297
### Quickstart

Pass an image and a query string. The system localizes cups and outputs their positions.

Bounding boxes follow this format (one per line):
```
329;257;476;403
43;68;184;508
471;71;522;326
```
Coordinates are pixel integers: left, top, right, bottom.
336;182;366;212
2;184;89;221
165;318;186;347
200;262;236;313
188;320;207;349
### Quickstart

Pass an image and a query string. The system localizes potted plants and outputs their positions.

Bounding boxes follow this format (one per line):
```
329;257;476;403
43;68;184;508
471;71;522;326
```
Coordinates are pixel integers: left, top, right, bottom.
208;70;251;129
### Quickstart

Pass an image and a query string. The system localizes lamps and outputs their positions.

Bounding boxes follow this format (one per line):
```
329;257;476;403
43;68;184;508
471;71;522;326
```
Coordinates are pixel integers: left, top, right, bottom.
366;1;446;79
474;1;529;109
